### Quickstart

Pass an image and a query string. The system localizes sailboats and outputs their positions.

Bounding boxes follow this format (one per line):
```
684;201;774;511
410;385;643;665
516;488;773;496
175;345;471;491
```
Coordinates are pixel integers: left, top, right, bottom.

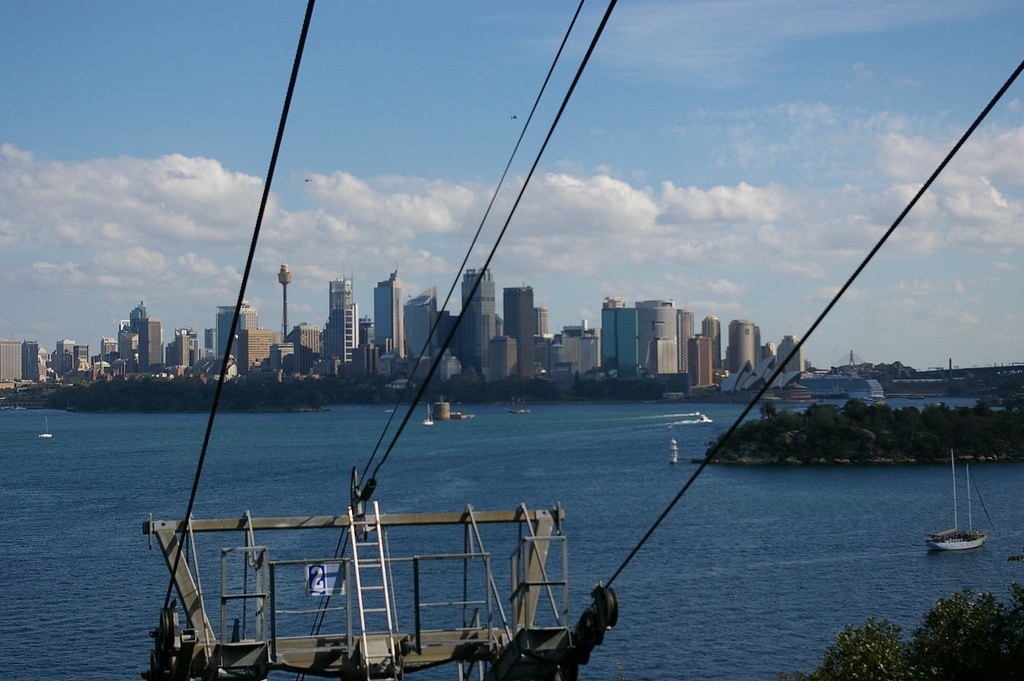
38;416;52;438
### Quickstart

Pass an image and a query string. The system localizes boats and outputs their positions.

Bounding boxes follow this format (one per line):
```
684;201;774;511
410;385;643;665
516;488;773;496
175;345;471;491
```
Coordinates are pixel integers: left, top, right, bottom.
907;393;924;399
921;448;991;550
509;393;530;414
696;415;712;424
422;403;434;425
